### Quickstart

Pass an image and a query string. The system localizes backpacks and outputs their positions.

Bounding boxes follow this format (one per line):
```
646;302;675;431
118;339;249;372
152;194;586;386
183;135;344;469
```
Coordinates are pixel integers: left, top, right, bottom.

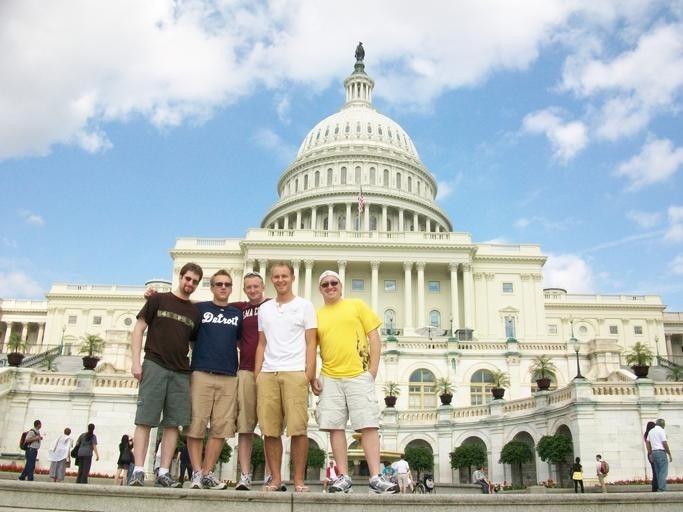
20;429;37;449
599;461;609;474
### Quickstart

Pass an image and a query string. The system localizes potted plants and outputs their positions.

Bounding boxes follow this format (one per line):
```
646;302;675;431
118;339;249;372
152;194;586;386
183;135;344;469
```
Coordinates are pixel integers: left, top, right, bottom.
432;376;457;404
7;332;28;365
78;333;105;369
623;342;653;377
487;370;511;399
382;381;400;407
529;354;558;392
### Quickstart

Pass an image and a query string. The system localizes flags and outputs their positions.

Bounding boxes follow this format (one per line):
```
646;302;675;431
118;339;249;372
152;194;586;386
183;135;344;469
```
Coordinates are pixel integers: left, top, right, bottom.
358;187;363;212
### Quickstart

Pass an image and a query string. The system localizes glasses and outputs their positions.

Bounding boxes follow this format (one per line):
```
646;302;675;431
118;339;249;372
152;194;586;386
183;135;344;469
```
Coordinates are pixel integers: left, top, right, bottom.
214;282;232;287
185;276;198;285
321;281;337;288
245;272;259;276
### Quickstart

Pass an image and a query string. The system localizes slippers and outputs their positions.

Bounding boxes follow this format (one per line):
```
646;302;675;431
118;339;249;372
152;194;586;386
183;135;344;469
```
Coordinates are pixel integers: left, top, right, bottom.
294;486;309;492
262;485;278;490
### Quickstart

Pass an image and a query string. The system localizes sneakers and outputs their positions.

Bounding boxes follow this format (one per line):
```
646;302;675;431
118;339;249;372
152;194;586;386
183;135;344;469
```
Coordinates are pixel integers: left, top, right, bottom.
236;472;253;490
190;470;227;490
154;473;182;488
368;473;400;493
327;473;353;494
265;474;286;491
129;471;145;486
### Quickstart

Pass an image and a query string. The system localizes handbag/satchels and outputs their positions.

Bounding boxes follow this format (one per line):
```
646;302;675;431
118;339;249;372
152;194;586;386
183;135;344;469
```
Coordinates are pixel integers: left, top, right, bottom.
120;443;130;463
571;471;584;481
70;446;78;458
426;478;434;488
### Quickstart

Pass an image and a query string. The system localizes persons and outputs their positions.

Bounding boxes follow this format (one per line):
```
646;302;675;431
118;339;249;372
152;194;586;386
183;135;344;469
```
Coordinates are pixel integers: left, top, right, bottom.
481;467;495;494
144;269;243;489
254;262;318;493
572;457;584;493
646;419;672;491
383;453;416;494
322;461;339;494
596;454;607;493
310;270;400;495
129;263;203;488
228;272;288;492
19;419;193;489
643;422;659;492
473;465;489;494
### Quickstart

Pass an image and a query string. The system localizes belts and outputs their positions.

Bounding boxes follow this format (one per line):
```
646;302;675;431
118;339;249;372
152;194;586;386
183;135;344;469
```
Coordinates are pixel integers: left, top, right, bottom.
651;450;664;454
